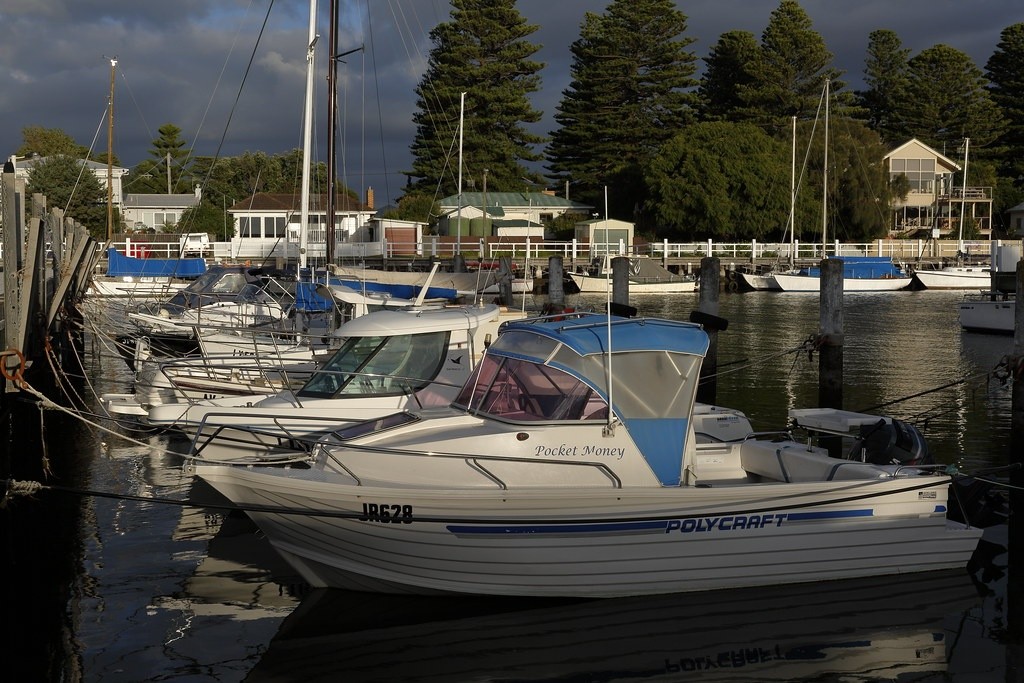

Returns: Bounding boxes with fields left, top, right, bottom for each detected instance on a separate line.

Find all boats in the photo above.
left=567, top=254, right=696, bottom=293
left=958, top=239, right=1021, bottom=335
left=190, top=314, right=984, bottom=601
left=1, top=0, right=759, bottom=455
left=236, top=566, right=983, bottom=683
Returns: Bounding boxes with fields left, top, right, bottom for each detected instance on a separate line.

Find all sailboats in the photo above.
left=773, top=78, right=914, bottom=293
left=912, top=136, right=1011, bottom=290
left=739, top=116, right=854, bottom=292
